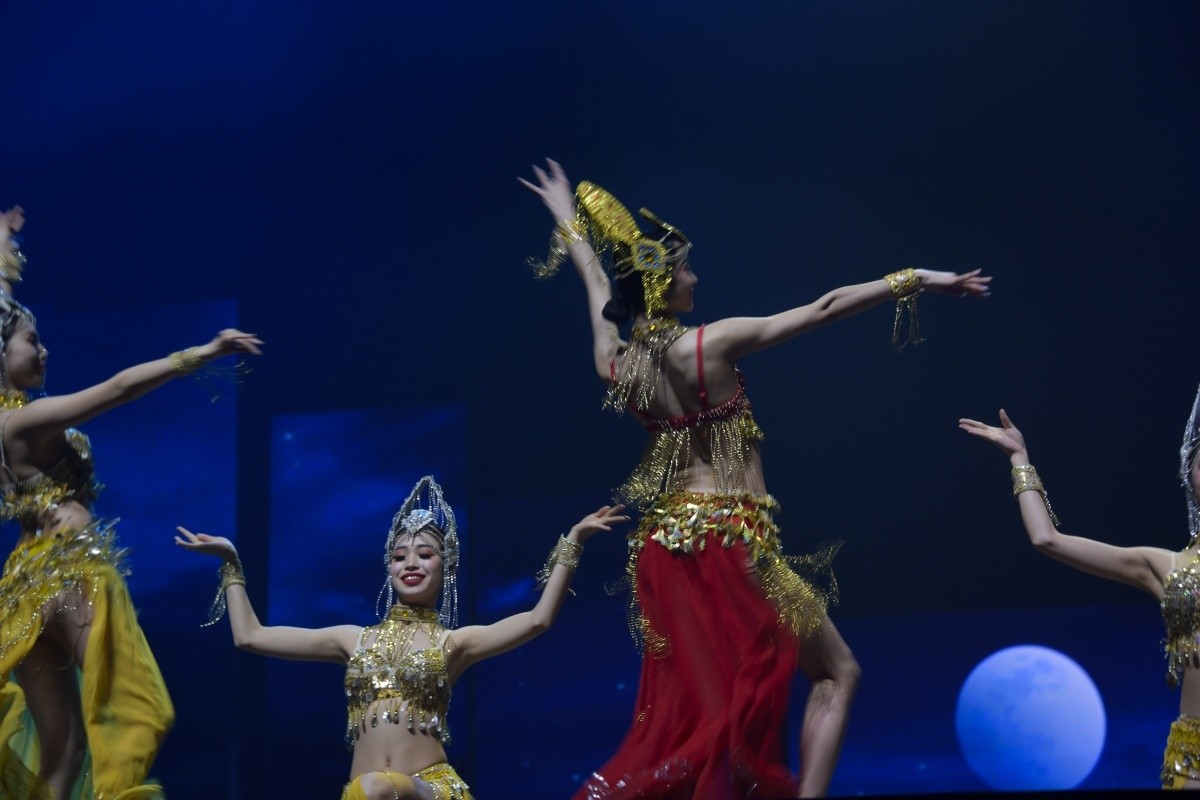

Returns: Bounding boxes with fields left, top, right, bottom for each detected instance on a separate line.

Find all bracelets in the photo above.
left=1011, top=464, right=1045, bottom=501
left=221, top=559, right=246, bottom=588
left=885, top=268, right=920, bottom=300
left=560, top=221, right=587, bottom=246
left=171, top=347, right=203, bottom=376
left=556, top=538, right=584, bottom=571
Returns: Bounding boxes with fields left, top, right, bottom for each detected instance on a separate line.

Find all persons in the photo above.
left=517, top=158, right=992, bottom=800
left=0, top=207, right=261, bottom=800
left=175, top=475, right=631, bottom=800
left=959, top=389, right=1200, bottom=789
